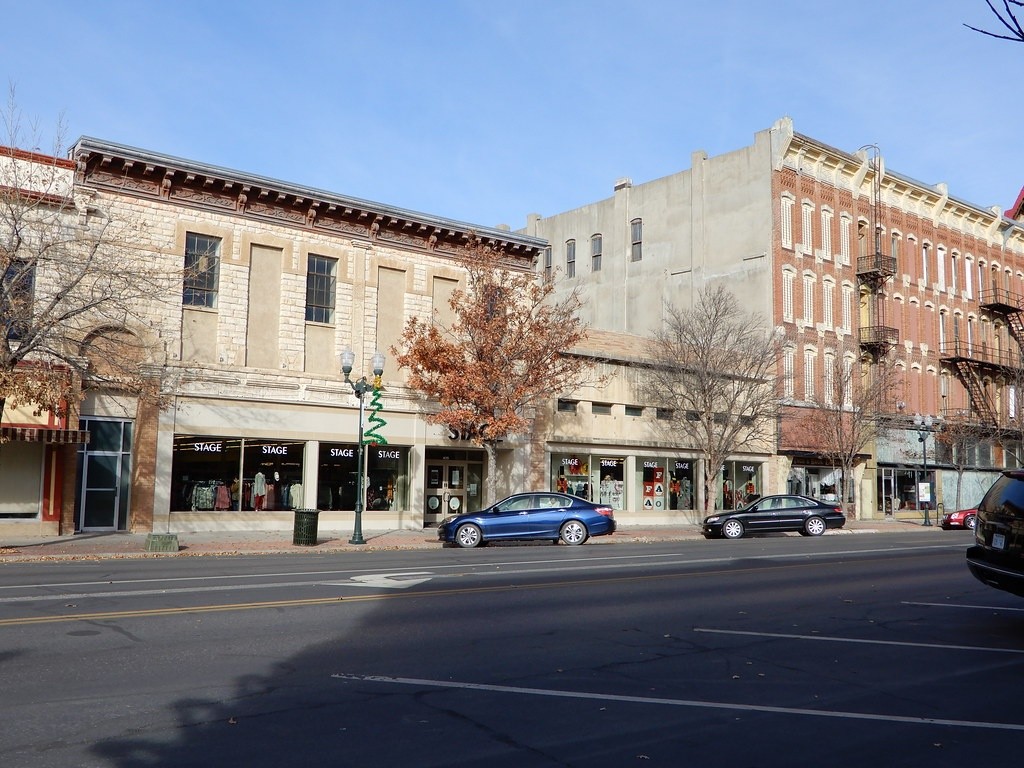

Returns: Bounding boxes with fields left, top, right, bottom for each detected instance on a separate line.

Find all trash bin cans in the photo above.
left=292, top=507, right=322, bottom=547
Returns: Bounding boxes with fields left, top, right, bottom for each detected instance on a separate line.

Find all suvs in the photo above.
left=965, top=470, right=1024, bottom=596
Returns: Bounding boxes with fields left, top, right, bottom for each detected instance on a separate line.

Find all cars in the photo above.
left=700, top=492, right=847, bottom=539
left=943, top=501, right=986, bottom=529
left=437, top=490, right=617, bottom=548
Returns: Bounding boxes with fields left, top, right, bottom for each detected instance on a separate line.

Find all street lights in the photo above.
left=914, top=413, right=937, bottom=527
left=337, top=343, right=387, bottom=544
